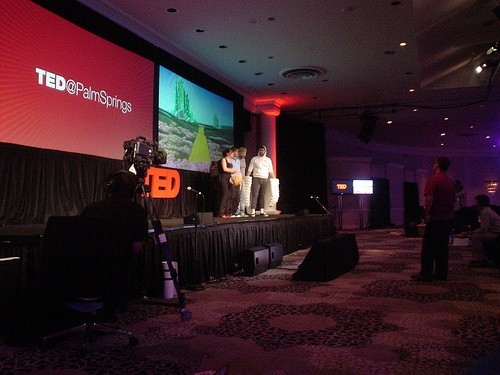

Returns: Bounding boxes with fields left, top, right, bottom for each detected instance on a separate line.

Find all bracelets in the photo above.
left=424, top=212, right=431, bottom=216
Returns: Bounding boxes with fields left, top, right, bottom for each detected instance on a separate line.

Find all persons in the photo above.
left=80, top=170, right=163, bottom=291
left=466, top=194, right=500, bottom=264
left=454, top=180, right=466, bottom=231
left=409, top=155, right=456, bottom=281
left=217, top=145, right=276, bottom=219
left=124, top=136, right=153, bottom=176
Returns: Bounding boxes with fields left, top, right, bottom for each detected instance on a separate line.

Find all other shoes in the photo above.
left=220, top=212, right=268, bottom=218
left=433, top=274, right=447, bottom=281
left=411, top=274, right=432, bottom=281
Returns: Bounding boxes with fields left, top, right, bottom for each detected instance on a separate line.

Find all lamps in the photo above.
left=475, top=61, right=498, bottom=74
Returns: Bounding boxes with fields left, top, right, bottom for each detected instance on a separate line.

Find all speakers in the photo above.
left=262, top=242, right=283, bottom=268
left=292, top=233, right=360, bottom=281
left=243, top=245, right=270, bottom=275
left=45, top=216, right=80, bottom=231
left=183, top=211, right=214, bottom=224
left=295, top=209, right=309, bottom=216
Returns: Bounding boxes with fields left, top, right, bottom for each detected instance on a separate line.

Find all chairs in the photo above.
left=39, top=216, right=149, bottom=358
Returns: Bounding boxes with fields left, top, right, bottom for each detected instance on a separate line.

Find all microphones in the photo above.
left=187, top=186, right=204, bottom=197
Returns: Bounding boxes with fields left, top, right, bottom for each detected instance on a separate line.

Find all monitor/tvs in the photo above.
left=330, top=179, right=353, bottom=195
left=353, top=179, right=374, bottom=195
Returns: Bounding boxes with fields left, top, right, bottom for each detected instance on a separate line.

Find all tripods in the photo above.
left=132, top=185, right=192, bottom=322
